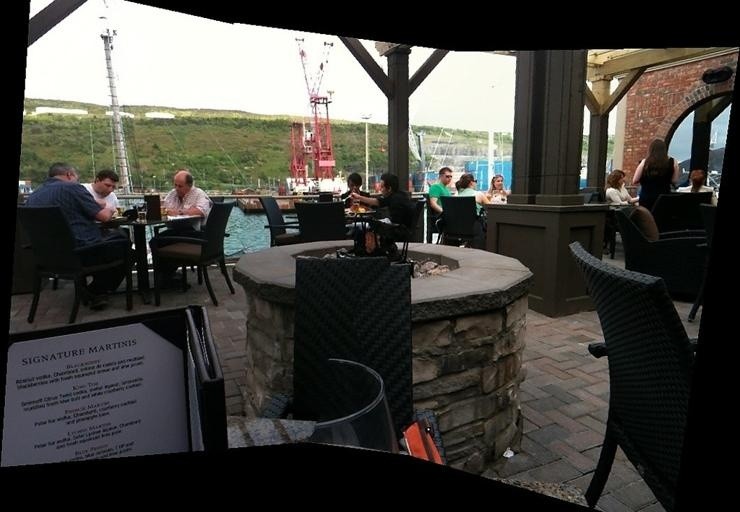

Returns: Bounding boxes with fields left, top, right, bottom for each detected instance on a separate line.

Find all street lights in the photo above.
left=360, top=114, right=373, bottom=194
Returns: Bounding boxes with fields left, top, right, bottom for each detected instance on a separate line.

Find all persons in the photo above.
left=25, top=162, right=137, bottom=311
left=341, top=172, right=370, bottom=208
left=149, top=170, right=217, bottom=293
left=430, top=167, right=510, bottom=249
left=353, top=174, right=415, bottom=242
left=316, top=193, right=354, bottom=210
left=75, top=170, right=132, bottom=247
left=605, top=138, right=718, bottom=211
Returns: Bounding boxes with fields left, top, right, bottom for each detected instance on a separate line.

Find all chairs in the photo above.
left=15, top=197, right=237, bottom=324
left=293, top=255, right=413, bottom=454
left=259, top=194, right=487, bottom=250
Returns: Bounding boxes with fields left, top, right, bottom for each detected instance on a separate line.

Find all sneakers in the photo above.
left=81, top=285, right=112, bottom=311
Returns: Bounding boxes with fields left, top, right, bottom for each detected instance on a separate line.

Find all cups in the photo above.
left=160, top=206, right=167, bottom=221
left=112, top=199, right=146, bottom=223
